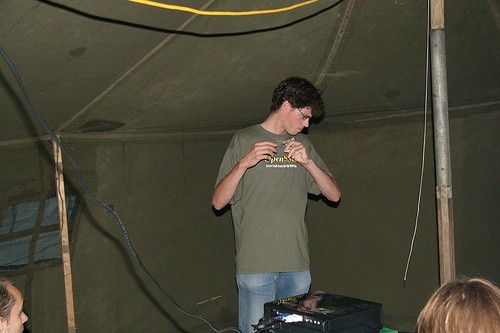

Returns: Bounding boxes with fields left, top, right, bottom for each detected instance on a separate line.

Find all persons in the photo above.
left=416, top=277, right=500, bottom=333
left=0, top=277, right=28, bottom=333
left=212, top=76, right=342, bottom=333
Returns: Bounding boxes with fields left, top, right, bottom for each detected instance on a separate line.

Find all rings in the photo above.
left=291, top=138, right=296, bottom=141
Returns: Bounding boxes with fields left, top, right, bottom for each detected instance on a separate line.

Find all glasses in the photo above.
left=297, top=108, right=313, bottom=120
